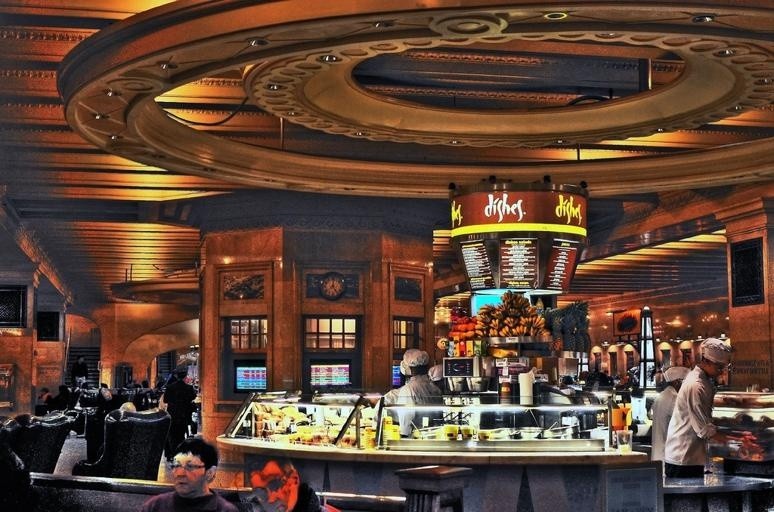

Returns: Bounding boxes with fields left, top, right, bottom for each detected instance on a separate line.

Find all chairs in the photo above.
left=0, top=387, right=172, bottom=481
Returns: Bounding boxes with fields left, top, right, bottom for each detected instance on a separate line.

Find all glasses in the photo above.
left=167, top=463, right=206, bottom=472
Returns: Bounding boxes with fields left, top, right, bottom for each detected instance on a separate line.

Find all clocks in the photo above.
left=317, top=271, right=347, bottom=301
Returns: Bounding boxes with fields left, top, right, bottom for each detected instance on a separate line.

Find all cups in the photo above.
left=519, top=373, right=534, bottom=396
left=476, top=430, right=490, bottom=440
left=444, top=424, right=460, bottom=440
left=500, top=396, right=511, bottom=405
left=520, top=396, right=533, bottom=405
left=500, top=382, right=511, bottom=396
left=460, top=426, right=476, bottom=438
left=617, top=430, right=632, bottom=452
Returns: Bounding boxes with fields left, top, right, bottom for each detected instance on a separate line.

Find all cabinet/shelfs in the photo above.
left=222, top=390, right=662, bottom=452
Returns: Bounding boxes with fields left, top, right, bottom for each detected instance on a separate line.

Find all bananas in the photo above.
left=478, top=289, right=550, bottom=337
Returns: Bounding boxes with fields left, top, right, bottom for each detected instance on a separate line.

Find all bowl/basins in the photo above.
left=446, top=377, right=466, bottom=392
left=487, top=425, right=579, bottom=439
left=466, top=376, right=490, bottom=391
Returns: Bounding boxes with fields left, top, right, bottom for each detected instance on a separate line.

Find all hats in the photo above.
left=664, top=366, right=692, bottom=382
left=701, top=338, right=730, bottom=365
left=400, top=349, right=443, bottom=381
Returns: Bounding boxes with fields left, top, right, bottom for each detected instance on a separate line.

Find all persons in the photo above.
left=373, top=348, right=443, bottom=428
left=663, top=337, right=734, bottom=478
left=241, top=452, right=327, bottom=512
left=163, top=367, right=196, bottom=461
left=36, top=387, right=54, bottom=404
left=651, top=366, right=692, bottom=461
left=71, top=356, right=88, bottom=388
left=137, top=438, right=239, bottom=512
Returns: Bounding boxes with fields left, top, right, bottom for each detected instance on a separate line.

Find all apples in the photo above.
left=445, top=315, right=475, bottom=341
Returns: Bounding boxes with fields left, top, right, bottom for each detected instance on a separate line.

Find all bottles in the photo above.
left=535, top=297, right=544, bottom=317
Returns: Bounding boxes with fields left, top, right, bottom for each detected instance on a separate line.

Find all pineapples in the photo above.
left=539, top=299, right=593, bottom=352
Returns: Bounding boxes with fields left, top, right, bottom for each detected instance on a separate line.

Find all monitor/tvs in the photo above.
left=442, top=357, right=475, bottom=378
left=308, top=359, right=352, bottom=388
left=233, top=358, right=267, bottom=394
left=391, top=364, right=402, bottom=389
